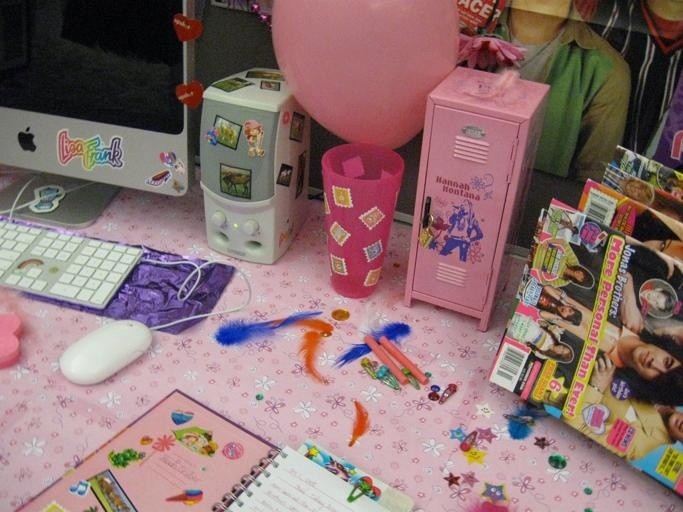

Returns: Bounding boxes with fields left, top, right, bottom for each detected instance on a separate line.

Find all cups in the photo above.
left=321, top=145, right=405, bottom=300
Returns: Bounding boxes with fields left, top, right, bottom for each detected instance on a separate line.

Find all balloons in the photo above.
left=273, top=0, right=462, bottom=148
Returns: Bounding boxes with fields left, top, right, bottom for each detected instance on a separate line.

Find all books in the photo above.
left=489, top=145, right=683, bottom=497
left=13, top=388, right=415, bottom=512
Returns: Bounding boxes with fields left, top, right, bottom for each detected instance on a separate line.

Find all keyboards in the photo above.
left=0, top=220, right=142, bottom=311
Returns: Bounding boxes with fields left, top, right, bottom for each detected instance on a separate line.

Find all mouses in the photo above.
left=59, top=318, right=154, bottom=387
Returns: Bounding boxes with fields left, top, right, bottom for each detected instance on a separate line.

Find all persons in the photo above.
left=243, top=120, right=265, bottom=159
left=483, top=0, right=632, bottom=184
left=439, top=200, right=483, bottom=261
left=578, top=0, right=683, bottom=171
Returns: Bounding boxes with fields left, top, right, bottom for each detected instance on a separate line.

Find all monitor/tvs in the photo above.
left=1, top=0, right=197, bottom=230
